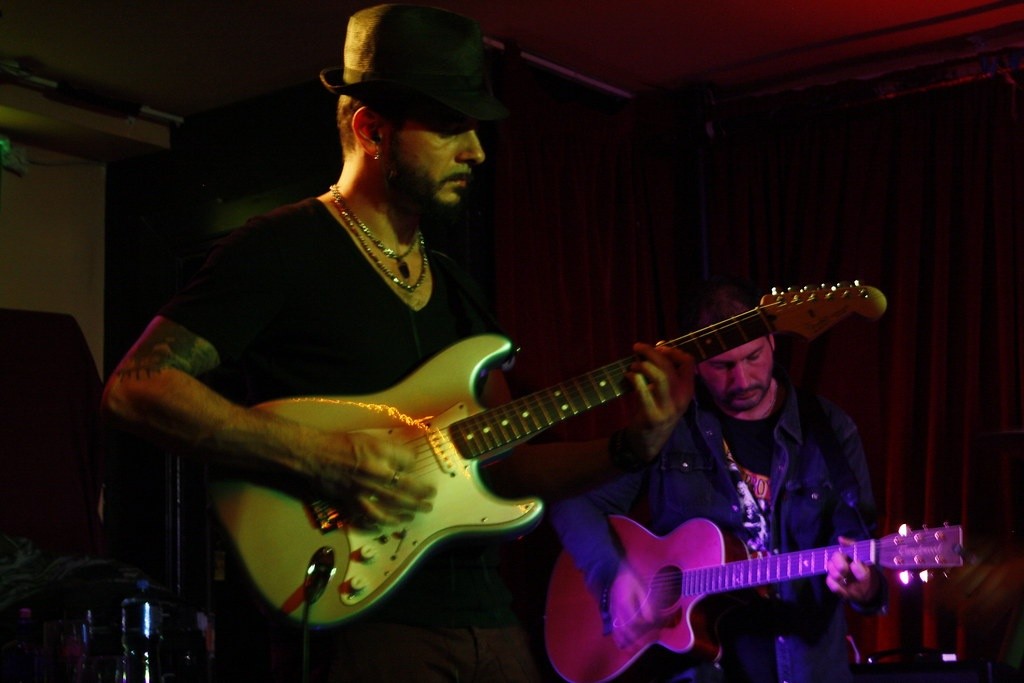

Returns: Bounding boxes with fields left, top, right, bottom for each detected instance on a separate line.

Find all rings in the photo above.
left=391, top=471, right=400, bottom=485
left=843, top=577, right=848, bottom=585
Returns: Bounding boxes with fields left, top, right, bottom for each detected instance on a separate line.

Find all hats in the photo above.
left=319, top=3, right=511, bottom=121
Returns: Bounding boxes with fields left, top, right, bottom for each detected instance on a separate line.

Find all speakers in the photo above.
left=846, top=660, right=1016, bottom=683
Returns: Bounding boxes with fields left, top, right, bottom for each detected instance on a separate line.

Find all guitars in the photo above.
left=205, top=280, right=891, bottom=628
left=542, top=506, right=966, bottom=683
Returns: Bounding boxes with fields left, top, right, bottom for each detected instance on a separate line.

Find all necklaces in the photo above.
left=330, top=184, right=428, bottom=292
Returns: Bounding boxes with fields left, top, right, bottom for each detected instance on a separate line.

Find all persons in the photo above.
left=549, top=272, right=890, bottom=683
left=101, top=0, right=693, bottom=683
